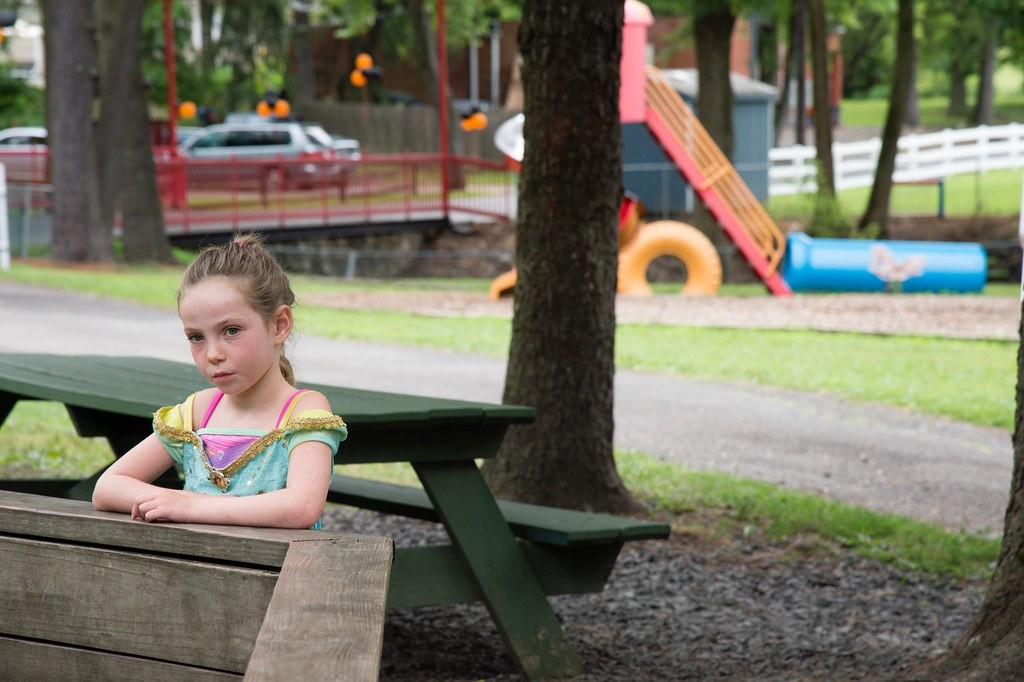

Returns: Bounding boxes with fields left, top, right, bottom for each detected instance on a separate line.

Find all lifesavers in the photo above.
left=616, top=220, right=723, bottom=300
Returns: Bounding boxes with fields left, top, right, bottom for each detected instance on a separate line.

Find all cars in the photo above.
left=146, top=122, right=205, bottom=164
left=295, top=124, right=363, bottom=197
left=151, top=121, right=339, bottom=194
left=0, top=124, right=56, bottom=184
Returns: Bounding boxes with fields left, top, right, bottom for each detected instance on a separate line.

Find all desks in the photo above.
left=0, top=354, right=673, bottom=682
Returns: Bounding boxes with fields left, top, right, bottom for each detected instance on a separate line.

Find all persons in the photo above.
left=85, top=237, right=348, bottom=524
